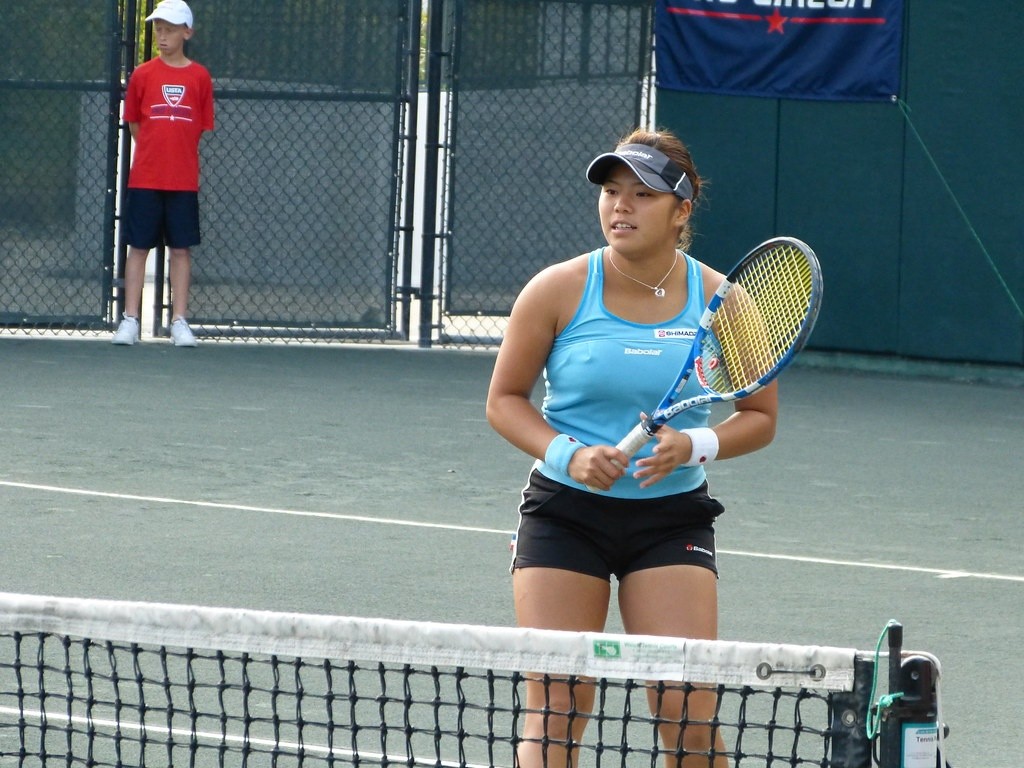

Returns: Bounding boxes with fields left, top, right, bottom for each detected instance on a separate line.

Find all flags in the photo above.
left=653, top=1, right=903, bottom=104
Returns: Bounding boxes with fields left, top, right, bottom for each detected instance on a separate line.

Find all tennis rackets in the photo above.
left=581, top=235, right=825, bottom=494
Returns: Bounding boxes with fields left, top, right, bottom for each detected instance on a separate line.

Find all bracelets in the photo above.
left=545, top=433, right=587, bottom=477
left=679, top=427, right=719, bottom=467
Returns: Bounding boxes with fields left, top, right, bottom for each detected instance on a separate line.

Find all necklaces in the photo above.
left=609, top=251, right=677, bottom=297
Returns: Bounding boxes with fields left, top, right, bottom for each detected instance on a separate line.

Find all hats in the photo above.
left=586, top=144, right=695, bottom=201
left=145, top=0, right=193, bottom=29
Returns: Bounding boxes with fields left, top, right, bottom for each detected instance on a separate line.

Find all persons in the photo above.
left=111, top=0, right=217, bottom=348
left=485, top=130, right=778, bottom=768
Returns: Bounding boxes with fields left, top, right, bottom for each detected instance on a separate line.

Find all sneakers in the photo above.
left=169, top=315, right=197, bottom=348
left=111, top=312, right=139, bottom=345
